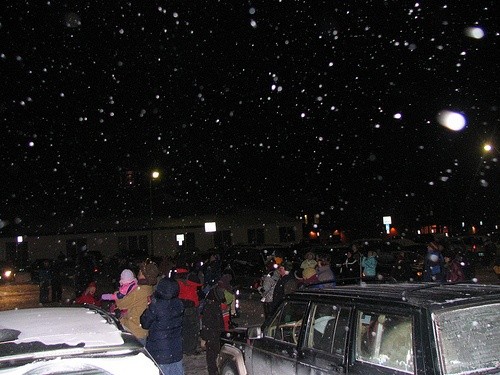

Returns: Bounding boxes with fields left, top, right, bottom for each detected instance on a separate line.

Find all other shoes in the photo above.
left=183, top=350, right=200, bottom=356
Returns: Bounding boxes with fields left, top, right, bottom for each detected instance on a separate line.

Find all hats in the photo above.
left=176, top=268, right=188, bottom=273
left=428, top=241, right=439, bottom=251
left=305, top=252, right=315, bottom=259
left=280, top=260, right=293, bottom=271
left=209, top=288, right=226, bottom=303
left=274, top=257, right=283, bottom=264
left=141, top=263, right=159, bottom=280
left=119, top=269, right=136, bottom=284
left=155, top=278, right=179, bottom=298
left=266, top=262, right=275, bottom=269
left=89, top=282, right=96, bottom=287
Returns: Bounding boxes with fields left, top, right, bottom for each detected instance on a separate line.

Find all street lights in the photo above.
left=149, top=171, right=159, bottom=256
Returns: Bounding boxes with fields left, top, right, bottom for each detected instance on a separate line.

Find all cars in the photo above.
left=221, top=234, right=444, bottom=292
left=217, top=280, right=500, bottom=375
left=0, top=303, right=163, bottom=375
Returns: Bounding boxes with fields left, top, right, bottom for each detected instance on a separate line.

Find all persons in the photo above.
left=140, top=278, right=184, bottom=375
left=39, top=233, right=500, bottom=375
